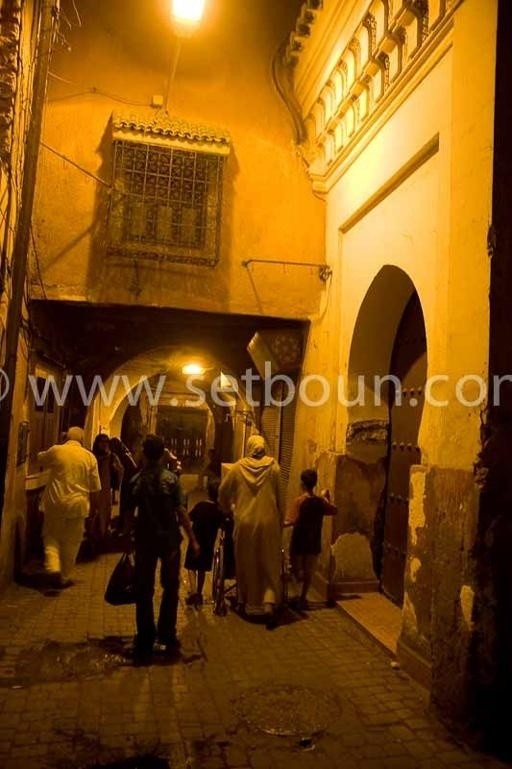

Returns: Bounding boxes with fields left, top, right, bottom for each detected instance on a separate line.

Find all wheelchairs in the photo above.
left=212, top=528, right=288, bottom=614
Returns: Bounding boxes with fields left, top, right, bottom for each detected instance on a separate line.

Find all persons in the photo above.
left=283, top=470, right=339, bottom=608
left=36, top=426, right=224, bottom=647
left=219, top=434, right=291, bottom=621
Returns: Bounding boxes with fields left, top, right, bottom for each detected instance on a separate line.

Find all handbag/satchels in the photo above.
left=104, top=552, right=135, bottom=605
